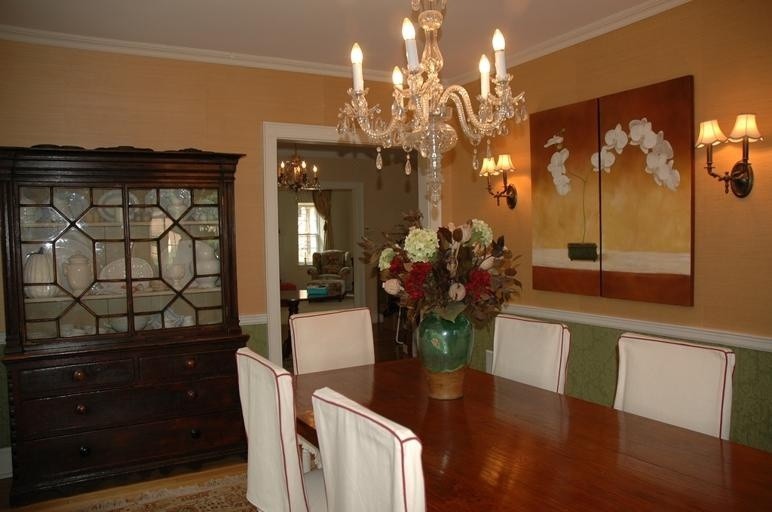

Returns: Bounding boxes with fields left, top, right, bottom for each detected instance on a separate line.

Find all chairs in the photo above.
left=235, top=347, right=329, bottom=512
left=489, top=314, right=572, bottom=397
left=289, top=307, right=381, bottom=472
left=613, top=331, right=738, bottom=441
left=311, top=383, right=428, bottom=512
left=307, top=249, right=353, bottom=300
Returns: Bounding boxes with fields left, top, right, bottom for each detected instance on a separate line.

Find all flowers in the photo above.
left=354, top=205, right=522, bottom=331
left=542, top=113, right=682, bottom=243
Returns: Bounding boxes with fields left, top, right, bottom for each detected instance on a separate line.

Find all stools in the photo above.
left=307, top=280, right=347, bottom=304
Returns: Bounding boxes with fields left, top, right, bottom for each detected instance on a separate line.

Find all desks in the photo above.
left=281, top=286, right=346, bottom=359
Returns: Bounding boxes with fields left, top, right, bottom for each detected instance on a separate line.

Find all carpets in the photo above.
left=68, top=470, right=258, bottom=512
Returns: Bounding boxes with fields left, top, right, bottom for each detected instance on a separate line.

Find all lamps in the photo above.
left=335, top=2, right=530, bottom=180
left=696, top=114, right=765, bottom=198
left=477, top=154, right=522, bottom=210
left=278, top=144, right=322, bottom=198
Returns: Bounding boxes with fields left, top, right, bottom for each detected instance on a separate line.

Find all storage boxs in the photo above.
left=309, top=288, right=329, bottom=297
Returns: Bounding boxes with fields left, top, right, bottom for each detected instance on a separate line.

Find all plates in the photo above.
left=99, top=258, right=153, bottom=295
left=159, top=239, right=219, bottom=289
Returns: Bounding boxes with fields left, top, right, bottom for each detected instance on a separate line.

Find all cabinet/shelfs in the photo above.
left=2, top=142, right=250, bottom=503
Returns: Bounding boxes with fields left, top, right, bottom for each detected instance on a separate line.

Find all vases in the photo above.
left=23, top=251, right=63, bottom=299
left=565, top=240, right=601, bottom=262
left=417, top=316, right=477, bottom=401
